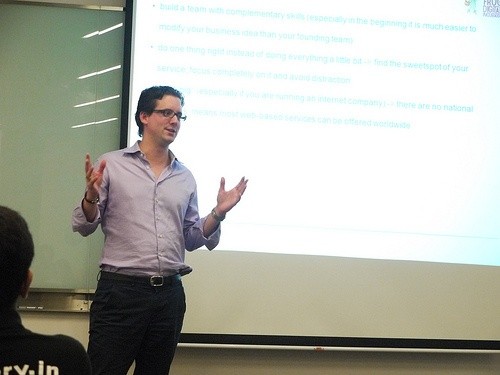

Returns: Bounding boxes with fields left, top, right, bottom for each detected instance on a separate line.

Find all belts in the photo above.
left=100, top=272, right=181, bottom=287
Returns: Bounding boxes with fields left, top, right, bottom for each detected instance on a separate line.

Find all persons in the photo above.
left=0, top=206, right=91, bottom=375
left=71, top=87, right=248, bottom=375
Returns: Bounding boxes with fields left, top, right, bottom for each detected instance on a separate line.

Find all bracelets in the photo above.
left=84, top=191, right=99, bottom=204
left=211, top=208, right=225, bottom=221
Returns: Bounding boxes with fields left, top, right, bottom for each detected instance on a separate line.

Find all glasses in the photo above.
left=153, top=109, right=182, bottom=120
